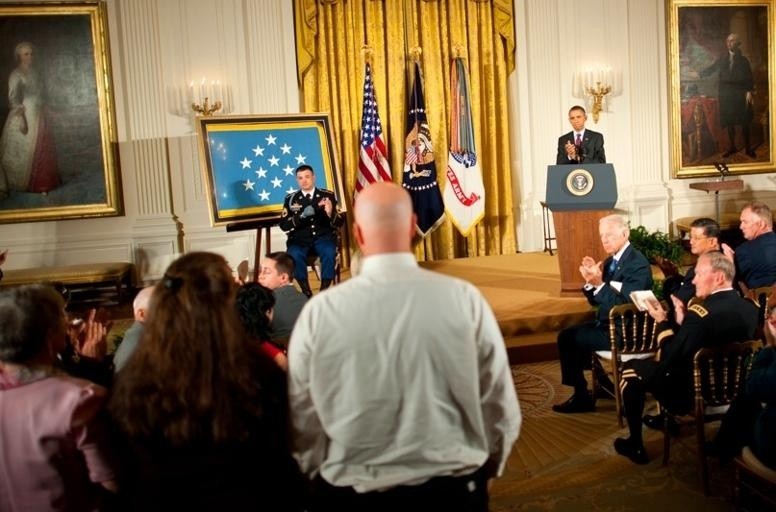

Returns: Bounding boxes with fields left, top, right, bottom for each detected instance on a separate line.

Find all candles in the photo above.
left=581, top=66, right=617, bottom=94
left=190, top=79, right=223, bottom=108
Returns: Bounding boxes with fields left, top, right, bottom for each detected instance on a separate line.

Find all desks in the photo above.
left=680, top=93, right=721, bottom=167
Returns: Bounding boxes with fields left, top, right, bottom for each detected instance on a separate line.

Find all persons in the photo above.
left=280, top=165, right=341, bottom=298
left=101, top=252, right=320, bottom=508
left=34, top=251, right=310, bottom=389
left=553, top=213, right=654, bottom=414
left=690, top=33, right=762, bottom=160
left=288, top=183, right=524, bottom=509
left=0, top=286, right=120, bottom=508
left=2, top=43, right=59, bottom=193
left=613, top=202, right=775, bottom=466
left=0, top=248, right=10, bottom=276
left=712, top=284, right=775, bottom=508
left=555, top=106, right=606, bottom=165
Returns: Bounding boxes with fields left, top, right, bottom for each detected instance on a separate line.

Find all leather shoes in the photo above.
left=614, top=438, right=650, bottom=464
left=643, top=414, right=664, bottom=431
left=552, top=394, right=596, bottom=412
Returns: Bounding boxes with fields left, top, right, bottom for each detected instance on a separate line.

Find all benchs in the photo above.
left=674, top=212, right=776, bottom=240
left=0, top=261, right=132, bottom=308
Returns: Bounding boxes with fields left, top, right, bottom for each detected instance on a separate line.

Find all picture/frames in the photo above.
left=664, top=2, right=776, bottom=180
left=194, top=112, right=350, bottom=228
left=0, top=1, right=126, bottom=225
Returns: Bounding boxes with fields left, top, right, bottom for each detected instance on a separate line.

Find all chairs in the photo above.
left=591, top=297, right=671, bottom=429
left=658, top=337, right=766, bottom=498
left=745, top=284, right=776, bottom=343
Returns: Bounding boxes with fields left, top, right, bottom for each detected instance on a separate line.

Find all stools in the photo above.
left=539, top=199, right=557, bottom=254
left=733, top=446, right=776, bottom=512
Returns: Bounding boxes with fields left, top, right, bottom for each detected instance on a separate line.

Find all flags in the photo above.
left=440, top=59, right=486, bottom=237
left=404, top=61, right=448, bottom=237
left=353, top=57, right=395, bottom=189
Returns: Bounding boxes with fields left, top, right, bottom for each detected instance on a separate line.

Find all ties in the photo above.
left=608, top=259, right=616, bottom=273
left=576, top=134, right=582, bottom=147
left=306, top=194, right=310, bottom=200
